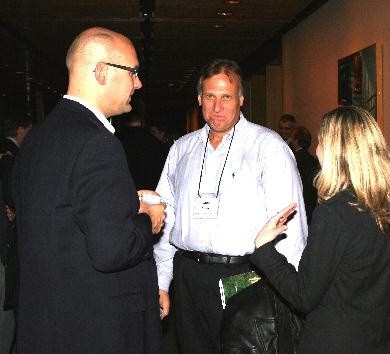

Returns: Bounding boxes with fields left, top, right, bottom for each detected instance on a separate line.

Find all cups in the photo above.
left=141, top=193, right=162, bottom=205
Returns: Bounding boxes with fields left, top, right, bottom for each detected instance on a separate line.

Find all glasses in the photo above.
left=93, top=63, right=141, bottom=79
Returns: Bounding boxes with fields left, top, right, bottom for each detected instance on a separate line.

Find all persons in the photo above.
left=11, top=26, right=167, bottom=354
left=250, top=107, right=390, bottom=354
left=0, top=98, right=320, bottom=312
left=150, top=60, right=308, bottom=354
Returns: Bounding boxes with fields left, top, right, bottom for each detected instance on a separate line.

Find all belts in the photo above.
left=180, top=250, right=249, bottom=263
